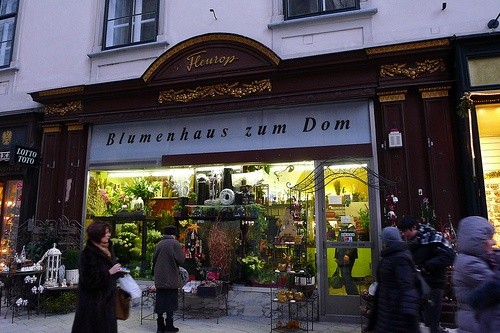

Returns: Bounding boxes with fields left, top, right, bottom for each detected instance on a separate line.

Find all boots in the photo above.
left=164, top=318, right=179, bottom=333
left=157, top=317, right=166, bottom=333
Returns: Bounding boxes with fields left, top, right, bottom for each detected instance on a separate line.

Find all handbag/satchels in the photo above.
left=330, top=267, right=340, bottom=289
left=119, top=274, right=142, bottom=299
left=416, top=275, right=431, bottom=300
left=116, top=286, right=132, bottom=320
left=179, top=266, right=189, bottom=286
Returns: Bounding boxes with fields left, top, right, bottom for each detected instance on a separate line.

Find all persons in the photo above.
left=364, top=226, right=424, bottom=333
left=71, top=220, right=131, bottom=333
left=399, top=220, right=497, bottom=333
left=334, top=215, right=359, bottom=295
left=452, top=216, right=500, bottom=333
left=154, top=226, right=186, bottom=333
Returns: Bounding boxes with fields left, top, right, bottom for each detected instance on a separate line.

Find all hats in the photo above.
left=164, top=226, right=179, bottom=235
left=380, top=227, right=404, bottom=242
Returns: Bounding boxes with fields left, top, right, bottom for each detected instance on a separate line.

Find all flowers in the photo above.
left=88, top=168, right=164, bottom=278
left=241, top=256, right=267, bottom=274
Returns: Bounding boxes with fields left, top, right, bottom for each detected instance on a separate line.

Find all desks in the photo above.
left=39, top=282, right=79, bottom=319
left=90, top=214, right=162, bottom=276
left=0, top=265, right=47, bottom=324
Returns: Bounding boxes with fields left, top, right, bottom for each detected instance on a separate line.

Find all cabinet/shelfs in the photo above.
left=268, top=241, right=320, bottom=333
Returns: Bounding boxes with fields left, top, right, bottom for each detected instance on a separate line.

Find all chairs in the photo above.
left=3, top=262, right=47, bottom=319
